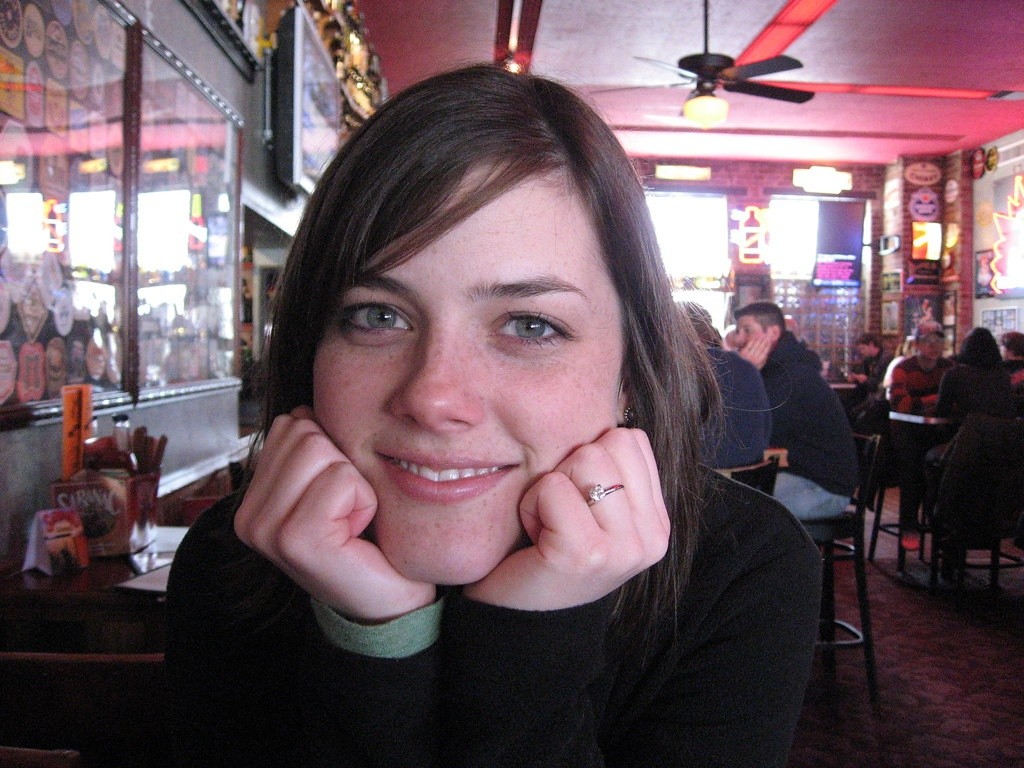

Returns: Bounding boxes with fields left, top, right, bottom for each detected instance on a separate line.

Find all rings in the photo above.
left=587, top=484, right=623, bottom=506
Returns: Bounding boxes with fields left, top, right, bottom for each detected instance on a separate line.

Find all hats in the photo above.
left=916, top=322, right=946, bottom=339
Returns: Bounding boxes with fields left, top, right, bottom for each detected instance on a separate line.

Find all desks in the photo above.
left=0, top=526, right=190, bottom=617
left=828, top=383, right=858, bottom=392
left=887, top=407, right=955, bottom=573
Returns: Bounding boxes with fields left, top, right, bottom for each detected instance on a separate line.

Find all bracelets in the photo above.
left=315, top=597, right=446, bottom=659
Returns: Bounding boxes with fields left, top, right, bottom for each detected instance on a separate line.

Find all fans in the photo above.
left=683, top=0, right=728, bottom=131
left=589, top=0, right=816, bottom=105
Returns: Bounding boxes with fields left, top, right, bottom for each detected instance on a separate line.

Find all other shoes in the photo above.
left=899, top=530, right=921, bottom=551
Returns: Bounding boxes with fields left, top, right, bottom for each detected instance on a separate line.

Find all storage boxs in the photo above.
left=48, top=471, right=160, bottom=553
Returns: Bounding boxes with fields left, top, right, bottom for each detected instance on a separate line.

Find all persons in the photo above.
left=846, top=332, right=894, bottom=416
left=881, top=321, right=1024, bottom=552
left=163, top=61, right=822, bottom=767
left=680, top=301, right=771, bottom=470
left=723, top=301, right=858, bottom=520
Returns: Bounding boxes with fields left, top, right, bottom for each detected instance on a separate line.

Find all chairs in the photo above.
left=0, top=649, right=165, bottom=768
left=725, top=387, right=1024, bottom=710
